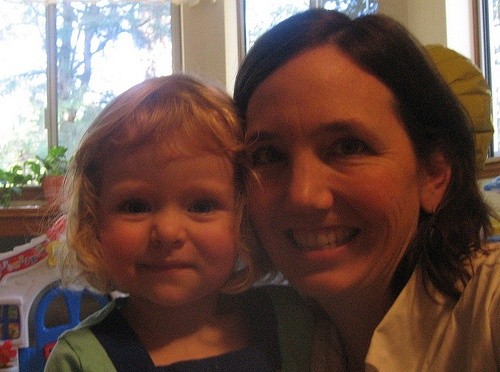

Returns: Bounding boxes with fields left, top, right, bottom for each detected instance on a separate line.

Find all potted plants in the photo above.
left=0, top=144, right=69, bottom=208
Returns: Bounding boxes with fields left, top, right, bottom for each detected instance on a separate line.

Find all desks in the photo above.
left=0, top=200, right=56, bottom=239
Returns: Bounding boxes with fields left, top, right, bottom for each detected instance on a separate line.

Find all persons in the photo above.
left=233, top=5, right=500, bottom=372
left=41, top=74, right=349, bottom=372
